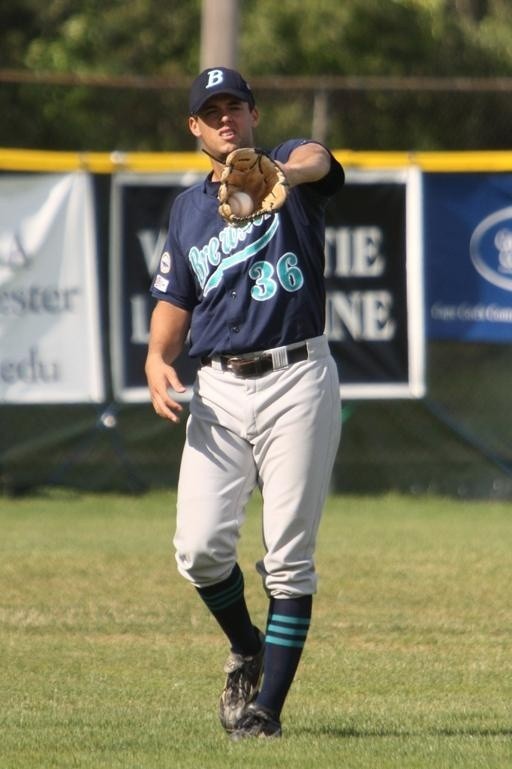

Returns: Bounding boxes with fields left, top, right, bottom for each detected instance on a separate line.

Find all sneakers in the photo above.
left=216, top=623, right=266, bottom=733
left=226, top=704, right=282, bottom=740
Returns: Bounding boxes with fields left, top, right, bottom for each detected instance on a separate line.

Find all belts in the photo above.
left=200, top=343, right=308, bottom=379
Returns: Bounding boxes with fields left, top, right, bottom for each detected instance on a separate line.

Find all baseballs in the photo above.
left=228, top=193, right=255, bottom=217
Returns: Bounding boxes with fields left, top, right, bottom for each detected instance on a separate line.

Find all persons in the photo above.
left=145, top=65, right=346, bottom=744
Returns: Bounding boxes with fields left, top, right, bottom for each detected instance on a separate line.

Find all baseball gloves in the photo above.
left=216, top=145, right=290, bottom=227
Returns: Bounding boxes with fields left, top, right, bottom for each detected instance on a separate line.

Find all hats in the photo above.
left=187, top=66, right=255, bottom=114
